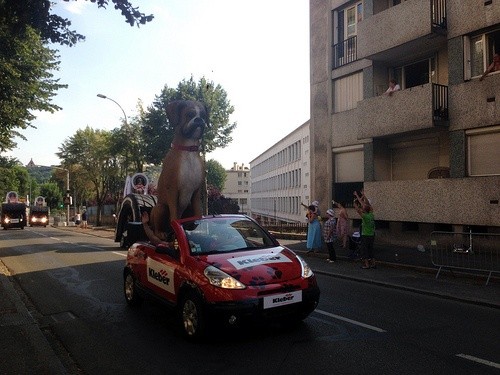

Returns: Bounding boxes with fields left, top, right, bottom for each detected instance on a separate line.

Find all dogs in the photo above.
left=149, top=99, right=208, bottom=241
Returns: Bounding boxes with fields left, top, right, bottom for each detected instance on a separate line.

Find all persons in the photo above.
left=384, top=80, right=401, bottom=96
left=353, top=191, right=377, bottom=269
left=74, top=212, right=81, bottom=231
left=323, top=209, right=337, bottom=263
left=301, top=200, right=323, bottom=256
left=80, top=211, right=89, bottom=231
left=332, top=200, right=348, bottom=249
left=142, top=211, right=195, bottom=250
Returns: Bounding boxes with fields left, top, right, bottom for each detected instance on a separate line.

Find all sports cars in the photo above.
left=122, top=213, right=321, bottom=339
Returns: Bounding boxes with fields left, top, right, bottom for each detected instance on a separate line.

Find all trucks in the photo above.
left=1, top=201, right=27, bottom=230
left=30, top=205, right=52, bottom=225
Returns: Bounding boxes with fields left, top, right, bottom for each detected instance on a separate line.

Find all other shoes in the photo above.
left=329, top=260, right=335, bottom=263
left=314, top=250, right=320, bottom=253
left=360, top=266, right=369, bottom=269
left=369, top=266, right=376, bottom=269
left=338, top=244, right=346, bottom=248
left=326, top=259, right=331, bottom=261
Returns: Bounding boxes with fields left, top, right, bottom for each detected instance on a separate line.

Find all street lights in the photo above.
left=97, top=93, right=129, bottom=178
left=52, top=165, right=73, bottom=226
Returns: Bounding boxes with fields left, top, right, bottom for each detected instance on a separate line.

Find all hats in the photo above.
left=312, top=201, right=319, bottom=206
left=327, top=209, right=335, bottom=217
left=352, top=232, right=361, bottom=238
